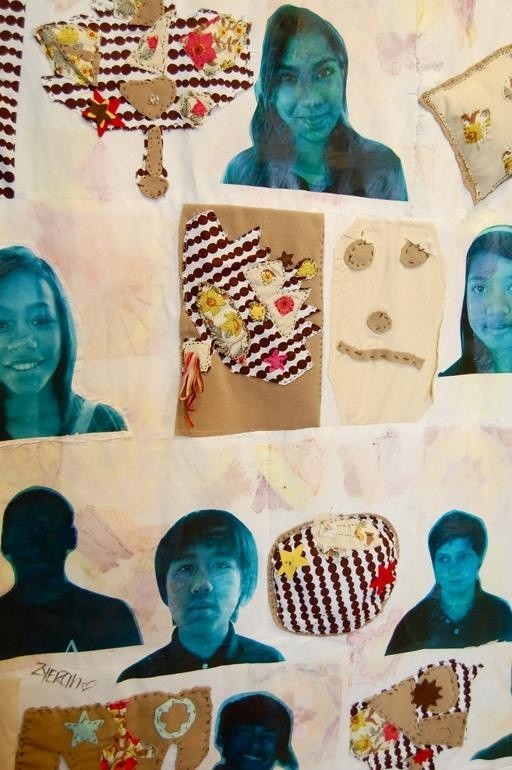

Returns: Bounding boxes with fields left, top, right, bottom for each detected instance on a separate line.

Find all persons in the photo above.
left=116, top=509, right=286, bottom=682
left=385, top=508, right=511, bottom=656
left=209, top=691, right=299, bottom=770
left=0, top=486, right=144, bottom=659
left=438, top=225, right=512, bottom=374
left=222, top=3, right=409, bottom=202
left=0, top=244, right=127, bottom=442
